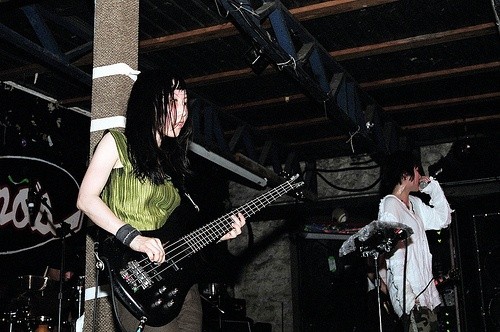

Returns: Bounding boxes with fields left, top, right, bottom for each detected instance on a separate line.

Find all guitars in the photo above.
left=97, top=173, right=304, bottom=327
left=434, top=267, right=462, bottom=286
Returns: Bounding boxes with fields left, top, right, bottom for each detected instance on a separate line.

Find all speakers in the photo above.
left=470, top=212, right=500, bottom=332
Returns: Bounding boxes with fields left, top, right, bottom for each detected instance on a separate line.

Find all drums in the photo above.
left=26, top=316, right=54, bottom=332
left=57, top=320, right=75, bottom=332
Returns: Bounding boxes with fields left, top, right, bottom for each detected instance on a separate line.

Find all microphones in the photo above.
left=31, top=188, right=47, bottom=201
left=420, top=168, right=443, bottom=189
